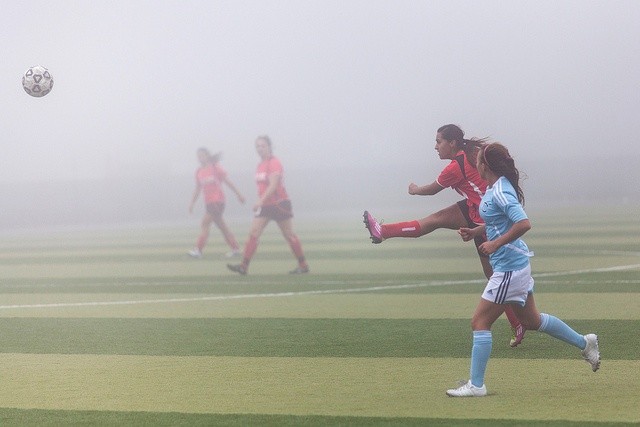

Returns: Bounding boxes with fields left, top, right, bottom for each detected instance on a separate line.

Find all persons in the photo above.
left=445, top=145, right=600, bottom=397
left=363, top=125, right=525, bottom=347
left=226, top=135, right=310, bottom=277
left=187, top=147, right=246, bottom=257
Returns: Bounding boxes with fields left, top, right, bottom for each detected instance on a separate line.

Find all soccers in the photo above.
left=23, top=66, right=53, bottom=97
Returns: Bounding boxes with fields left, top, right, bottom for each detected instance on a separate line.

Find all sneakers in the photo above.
left=226, top=262, right=247, bottom=275
left=363, top=211, right=385, bottom=244
left=289, top=264, right=309, bottom=274
left=580, top=334, right=601, bottom=373
left=224, top=248, right=242, bottom=258
left=509, top=323, right=526, bottom=347
left=187, top=247, right=203, bottom=258
left=445, top=381, right=487, bottom=398
